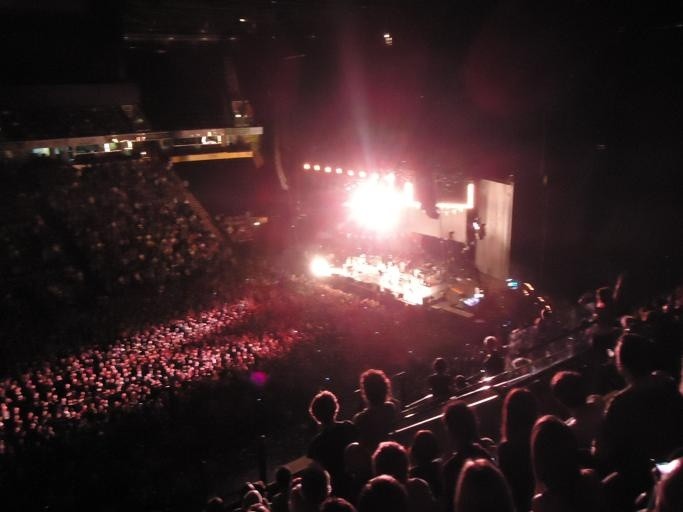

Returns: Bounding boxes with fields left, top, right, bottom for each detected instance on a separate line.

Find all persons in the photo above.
left=0, top=159, right=683, bottom=512
